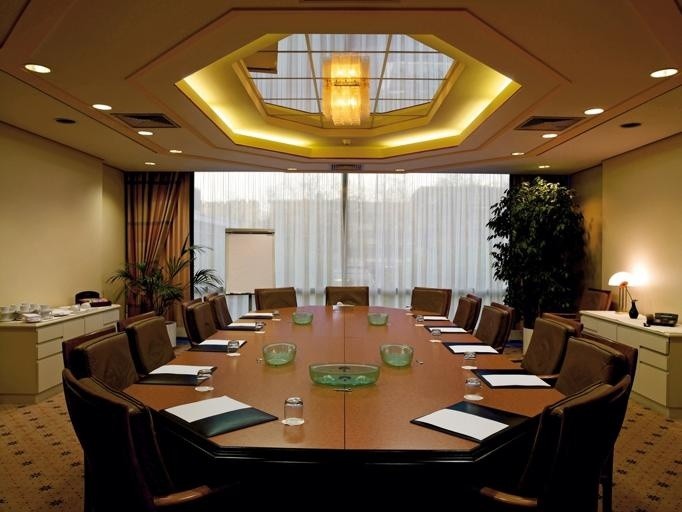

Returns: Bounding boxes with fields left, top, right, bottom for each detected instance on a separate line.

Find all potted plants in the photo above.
left=483, top=172, right=596, bottom=358
left=102, top=230, right=227, bottom=350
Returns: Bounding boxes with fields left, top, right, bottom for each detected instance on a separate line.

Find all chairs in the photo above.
left=181, top=298, right=219, bottom=346
left=116, top=309, right=178, bottom=376
left=203, top=292, right=232, bottom=328
left=255, top=286, right=297, bottom=310
left=410, top=286, right=453, bottom=320
left=478, top=330, right=639, bottom=512
left=510, top=310, right=584, bottom=389
left=325, top=285, right=370, bottom=307
left=59, top=366, right=224, bottom=512
left=452, top=292, right=482, bottom=335
left=60, top=325, right=139, bottom=393
left=474, top=301, right=516, bottom=353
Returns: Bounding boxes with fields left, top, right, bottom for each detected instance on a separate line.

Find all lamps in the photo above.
left=319, top=51, right=373, bottom=130
left=608, top=268, right=643, bottom=316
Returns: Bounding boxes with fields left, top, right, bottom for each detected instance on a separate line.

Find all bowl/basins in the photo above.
left=367, top=312, right=387, bottom=327
left=377, top=343, right=415, bottom=367
left=262, top=342, right=296, bottom=366
left=293, top=310, right=315, bottom=325
left=307, top=361, right=380, bottom=385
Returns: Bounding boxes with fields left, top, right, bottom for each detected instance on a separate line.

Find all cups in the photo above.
left=462, top=378, right=483, bottom=400
left=271, top=309, right=280, bottom=321
left=430, top=329, right=442, bottom=343
left=404, top=305, right=414, bottom=315
left=284, top=396, right=304, bottom=426
left=81, top=302, right=92, bottom=309
left=255, top=320, right=264, bottom=332
left=0, top=303, right=51, bottom=322
left=415, top=315, right=424, bottom=327
left=196, top=367, right=214, bottom=390
left=462, top=352, right=476, bottom=369
left=645, top=313, right=653, bottom=325
left=71, top=303, right=80, bottom=311
left=227, top=340, right=240, bottom=354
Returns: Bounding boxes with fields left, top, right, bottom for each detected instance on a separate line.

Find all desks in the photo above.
left=122, top=305, right=570, bottom=512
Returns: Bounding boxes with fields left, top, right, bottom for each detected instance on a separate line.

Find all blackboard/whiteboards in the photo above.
left=225, top=227, right=276, bottom=295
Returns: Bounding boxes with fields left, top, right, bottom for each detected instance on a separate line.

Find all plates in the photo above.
left=50, top=308, right=69, bottom=317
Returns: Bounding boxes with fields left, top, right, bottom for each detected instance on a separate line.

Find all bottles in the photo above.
left=667, top=318, right=674, bottom=324
left=655, top=317, right=661, bottom=322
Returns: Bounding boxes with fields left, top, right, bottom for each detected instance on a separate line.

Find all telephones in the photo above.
left=647, top=313, right=678, bottom=327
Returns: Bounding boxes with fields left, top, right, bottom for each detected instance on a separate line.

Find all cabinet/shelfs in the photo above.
left=578, top=309, right=682, bottom=422
left=1, top=303, right=122, bottom=408
